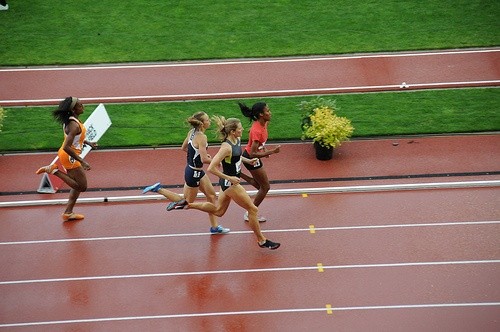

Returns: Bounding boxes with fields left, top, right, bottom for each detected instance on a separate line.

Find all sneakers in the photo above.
left=167, top=199, right=188, bottom=212
left=256, top=238, right=281, bottom=250
left=62, top=212, right=85, bottom=221
left=143, top=181, right=161, bottom=194
left=210, top=225, right=230, bottom=235
left=244, top=211, right=267, bottom=222
left=36, top=164, right=59, bottom=174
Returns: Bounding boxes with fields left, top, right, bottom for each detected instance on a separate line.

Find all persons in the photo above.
left=143, top=112, right=230, bottom=234
left=166, top=115, right=281, bottom=250
left=238, top=101, right=280, bottom=222
left=36, top=96, right=98, bottom=221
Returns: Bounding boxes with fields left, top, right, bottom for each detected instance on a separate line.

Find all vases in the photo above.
left=314, top=140, right=334, bottom=161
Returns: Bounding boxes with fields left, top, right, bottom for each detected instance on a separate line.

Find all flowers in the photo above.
left=301, top=107, right=354, bottom=149
